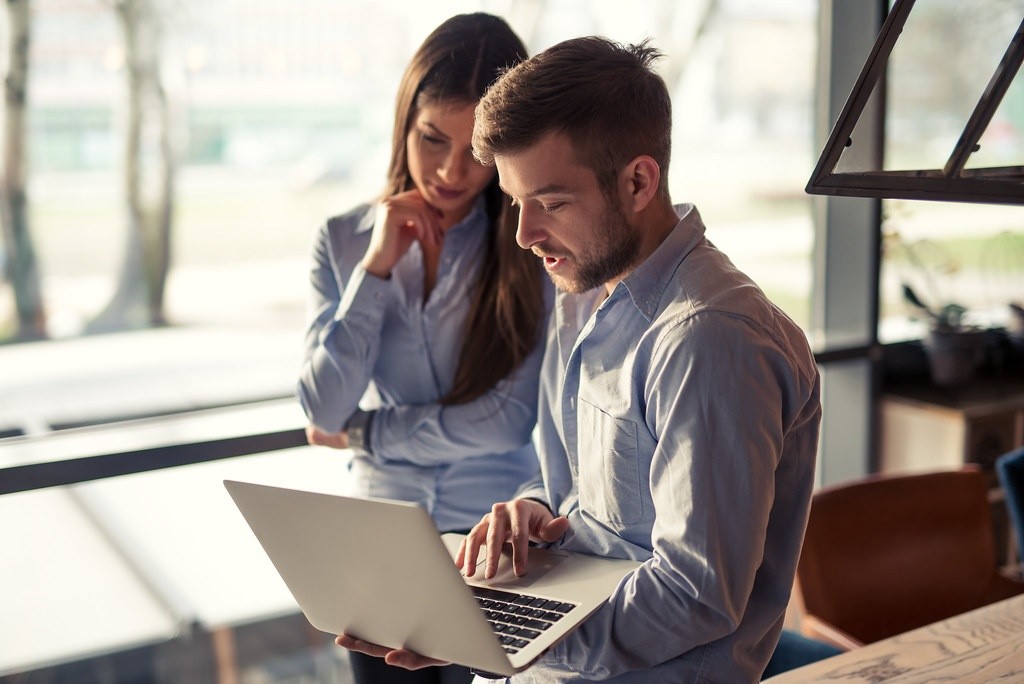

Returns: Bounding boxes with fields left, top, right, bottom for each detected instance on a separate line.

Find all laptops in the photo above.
left=223, top=480, right=645, bottom=677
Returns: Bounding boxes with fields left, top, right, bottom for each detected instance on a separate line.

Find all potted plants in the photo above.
left=904, top=281, right=980, bottom=389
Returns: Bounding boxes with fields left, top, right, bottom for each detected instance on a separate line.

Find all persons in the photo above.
left=335, top=36, right=821, bottom=684
left=292, top=11, right=558, bottom=684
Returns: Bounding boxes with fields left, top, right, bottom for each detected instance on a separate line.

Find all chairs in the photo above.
left=760, top=448, right=1024, bottom=680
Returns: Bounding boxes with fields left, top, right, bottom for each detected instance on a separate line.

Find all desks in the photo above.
left=879, top=393, right=1024, bottom=570
left=759, top=593, right=1024, bottom=684
